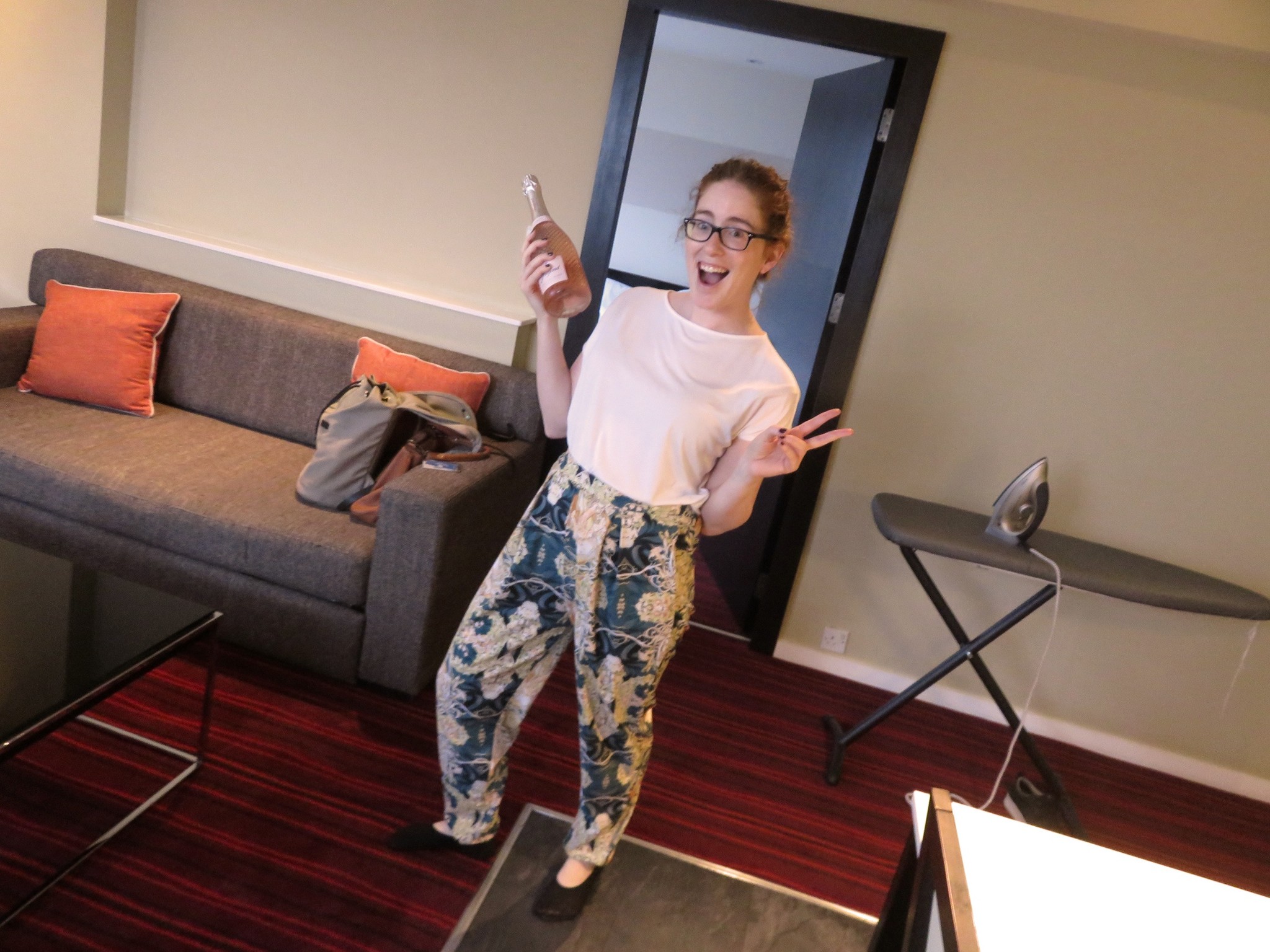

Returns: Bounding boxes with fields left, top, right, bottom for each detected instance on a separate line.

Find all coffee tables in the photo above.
left=0, top=540, right=224, bottom=930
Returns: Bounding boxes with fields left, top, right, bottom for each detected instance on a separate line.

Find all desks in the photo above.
left=868, top=786, right=1270, bottom=952
left=820, top=487, right=1270, bottom=836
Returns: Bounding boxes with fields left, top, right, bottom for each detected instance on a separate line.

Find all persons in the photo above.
left=388, top=160, right=853, bottom=920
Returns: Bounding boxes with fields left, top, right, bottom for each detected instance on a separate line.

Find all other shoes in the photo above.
left=389, top=821, right=503, bottom=860
left=536, top=856, right=603, bottom=919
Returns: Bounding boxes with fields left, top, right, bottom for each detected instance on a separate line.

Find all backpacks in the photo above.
left=297, top=372, right=484, bottom=511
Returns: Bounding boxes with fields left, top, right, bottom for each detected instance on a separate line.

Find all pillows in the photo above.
left=18, top=278, right=181, bottom=419
left=341, top=337, right=489, bottom=417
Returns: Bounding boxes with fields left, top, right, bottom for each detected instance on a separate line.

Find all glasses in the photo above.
left=684, top=217, right=779, bottom=252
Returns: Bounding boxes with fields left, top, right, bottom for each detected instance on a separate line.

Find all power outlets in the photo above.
left=821, top=626, right=850, bottom=654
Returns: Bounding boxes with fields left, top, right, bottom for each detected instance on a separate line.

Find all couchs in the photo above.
left=0, top=249, right=548, bottom=698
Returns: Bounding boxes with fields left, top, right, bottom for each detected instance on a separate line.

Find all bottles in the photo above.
left=519, top=173, right=592, bottom=316
